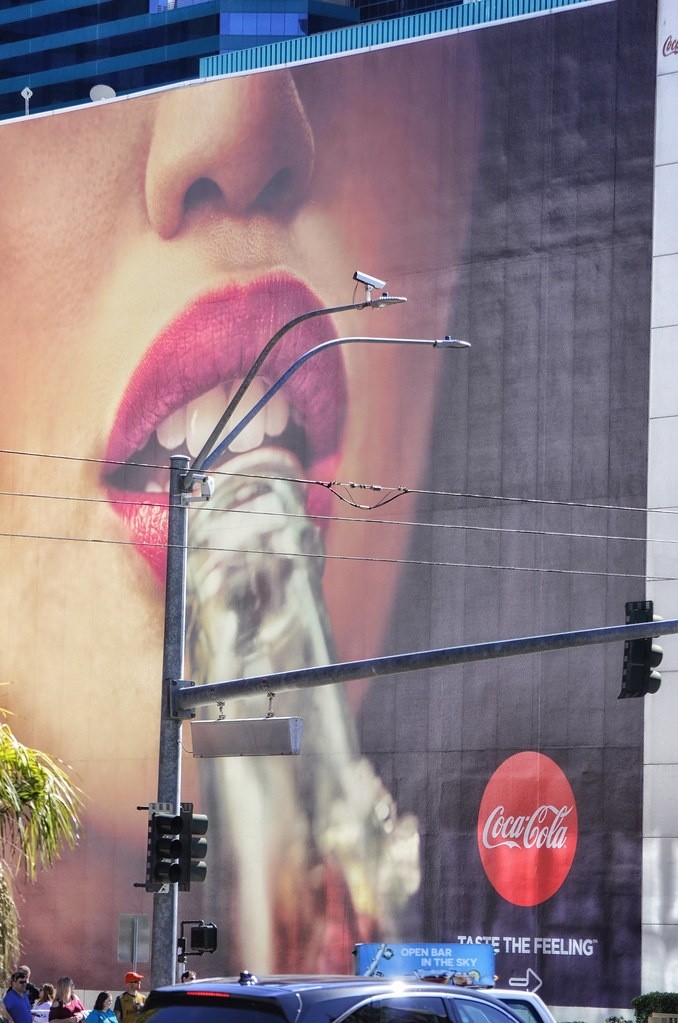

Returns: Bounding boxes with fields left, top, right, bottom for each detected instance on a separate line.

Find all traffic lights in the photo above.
left=617, top=600, right=663, bottom=699
left=191, top=923, right=218, bottom=952
left=146, top=803, right=183, bottom=894
left=178, top=803, right=208, bottom=892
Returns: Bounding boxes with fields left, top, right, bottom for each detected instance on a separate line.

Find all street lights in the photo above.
left=149, top=295, right=476, bottom=992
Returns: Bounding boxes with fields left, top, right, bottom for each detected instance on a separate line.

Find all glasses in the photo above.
left=188, top=978, right=196, bottom=980
left=40, top=989, right=44, bottom=992
left=14, top=980, right=27, bottom=984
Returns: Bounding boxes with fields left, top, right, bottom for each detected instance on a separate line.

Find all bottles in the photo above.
left=178, top=447, right=405, bottom=978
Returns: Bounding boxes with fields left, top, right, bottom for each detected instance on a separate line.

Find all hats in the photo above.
left=125, top=972, right=144, bottom=983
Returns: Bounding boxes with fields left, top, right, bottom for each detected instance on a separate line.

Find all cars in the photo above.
left=133, top=971, right=527, bottom=1023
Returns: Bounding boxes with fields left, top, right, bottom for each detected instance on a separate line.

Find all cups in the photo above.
left=413, top=968, right=456, bottom=984
left=453, top=973, right=475, bottom=986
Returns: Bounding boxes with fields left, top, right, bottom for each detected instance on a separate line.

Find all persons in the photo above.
left=7, top=962, right=201, bottom=1023
